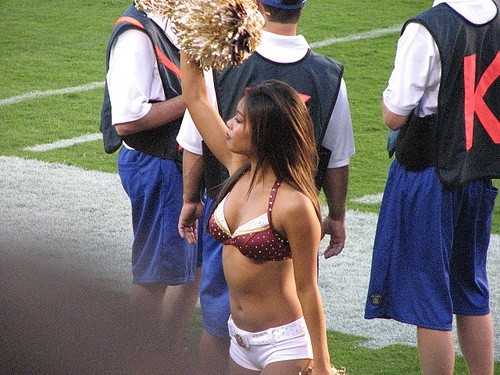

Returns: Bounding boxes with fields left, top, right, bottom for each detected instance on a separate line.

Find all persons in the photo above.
left=178, top=48, right=335, bottom=375
left=100, top=0, right=203, bottom=329
left=176, top=0, right=355, bottom=375
left=365, top=0, right=500, bottom=374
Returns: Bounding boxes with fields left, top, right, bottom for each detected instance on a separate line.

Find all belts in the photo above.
left=227, top=318, right=308, bottom=348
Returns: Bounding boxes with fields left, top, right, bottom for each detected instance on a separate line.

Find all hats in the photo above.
left=259, top=0, right=307, bottom=9
